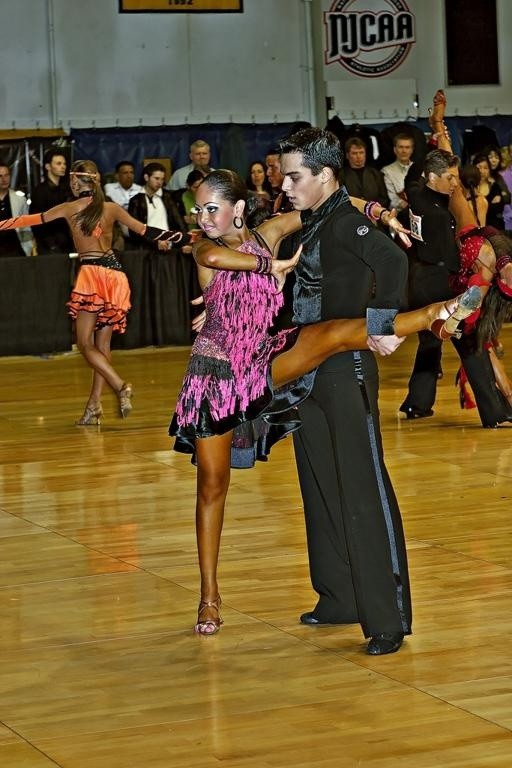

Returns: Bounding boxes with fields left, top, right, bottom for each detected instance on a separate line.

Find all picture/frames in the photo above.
left=118, top=0, right=243, bottom=14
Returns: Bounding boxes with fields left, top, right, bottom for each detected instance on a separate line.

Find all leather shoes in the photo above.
left=368, top=633, right=405, bottom=655
left=300, top=611, right=324, bottom=625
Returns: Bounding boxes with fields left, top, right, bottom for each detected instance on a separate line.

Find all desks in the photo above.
left=0, top=239, right=300, bottom=356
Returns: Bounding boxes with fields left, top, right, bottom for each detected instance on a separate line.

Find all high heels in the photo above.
left=195, top=593, right=223, bottom=635
left=76, top=405, right=104, bottom=425
left=406, top=406, right=434, bottom=419
left=428, top=90, right=447, bottom=129
left=430, top=286, right=484, bottom=342
left=118, top=381, right=133, bottom=419
left=489, top=418, right=512, bottom=431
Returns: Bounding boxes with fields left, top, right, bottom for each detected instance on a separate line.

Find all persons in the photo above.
left=168, top=168, right=482, bottom=635
left=190, top=128, right=413, bottom=656
left=428, top=88, right=511, bottom=409
left=0, top=132, right=512, bottom=256
left=1, top=160, right=199, bottom=426
left=399, top=149, right=511, bottom=428
